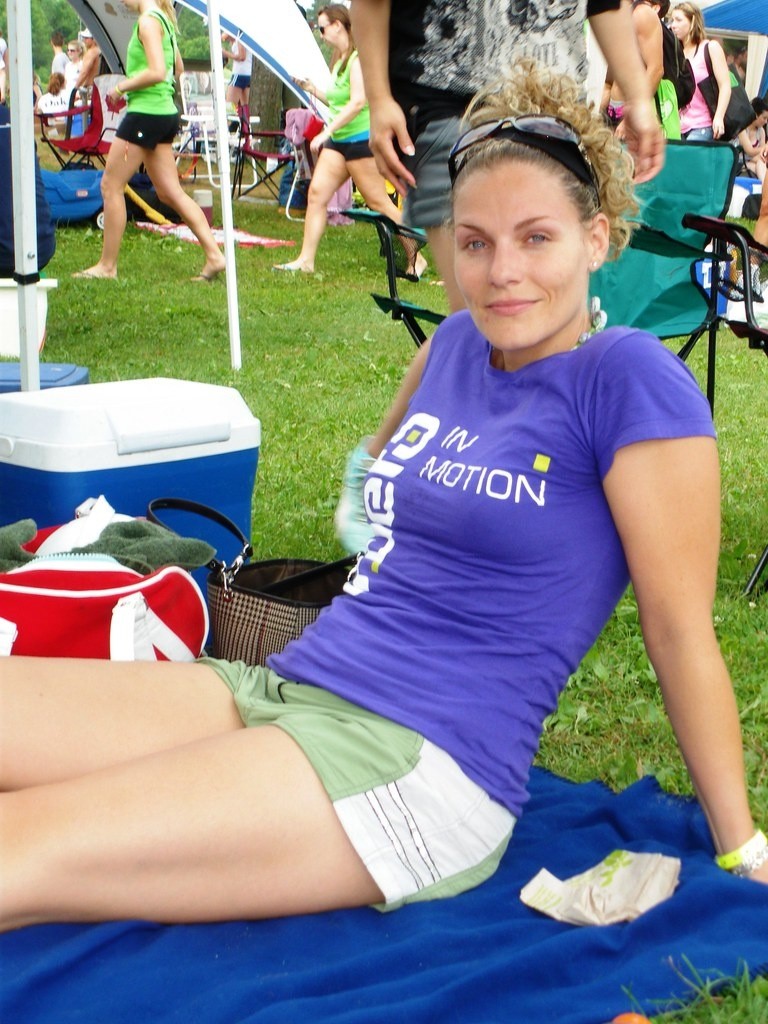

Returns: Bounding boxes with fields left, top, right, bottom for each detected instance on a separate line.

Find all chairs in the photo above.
left=39, top=74, right=134, bottom=170
left=230, top=107, right=318, bottom=203
left=338, top=142, right=767, bottom=427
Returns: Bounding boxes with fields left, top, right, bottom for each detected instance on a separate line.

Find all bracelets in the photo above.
left=733, top=853, right=767, bottom=879
left=115, top=83, right=125, bottom=96
left=713, top=827, right=767, bottom=869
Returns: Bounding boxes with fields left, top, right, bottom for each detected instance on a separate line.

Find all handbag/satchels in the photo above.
left=697, top=41, right=757, bottom=140
left=653, top=78, right=682, bottom=141
left=0, top=516, right=210, bottom=662
left=147, top=498, right=364, bottom=669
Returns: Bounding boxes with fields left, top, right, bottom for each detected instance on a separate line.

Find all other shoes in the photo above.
left=333, top=438, right=373, bottom=556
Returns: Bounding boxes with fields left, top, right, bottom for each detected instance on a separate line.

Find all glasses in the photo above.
left=67, top=49, right=78, bottom=52
left=320, top=22, right=335, bottom=35
left=81, top=28, right=93, bottom=38
left=447, top=113, right=603, bottom=216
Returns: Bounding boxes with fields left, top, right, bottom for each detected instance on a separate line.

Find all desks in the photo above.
left=174, top=114, right=261, bottom=188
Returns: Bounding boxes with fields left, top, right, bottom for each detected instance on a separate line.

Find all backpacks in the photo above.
left=663, top=24, right=695, bottom=109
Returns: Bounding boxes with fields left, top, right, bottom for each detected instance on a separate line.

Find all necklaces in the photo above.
left=573, top=296, right=607, bottom=353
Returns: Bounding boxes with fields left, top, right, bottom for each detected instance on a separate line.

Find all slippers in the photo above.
left=71, top=268, right=118, bottom=279
left=271, top=264, right=314, bottom=275
left=190, top=272, right=226, bottom=283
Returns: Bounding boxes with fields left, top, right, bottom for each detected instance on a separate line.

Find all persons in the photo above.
left=0, top=0, right=768, bottom=284
left=1, top=70, right=768, bottom=930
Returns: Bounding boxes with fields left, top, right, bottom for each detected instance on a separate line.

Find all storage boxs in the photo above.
left=0, top=276, right=59, bottom=359
left=0, top=377, right=263, bottom=649
left=0, top=362, right=91, bottom=395
